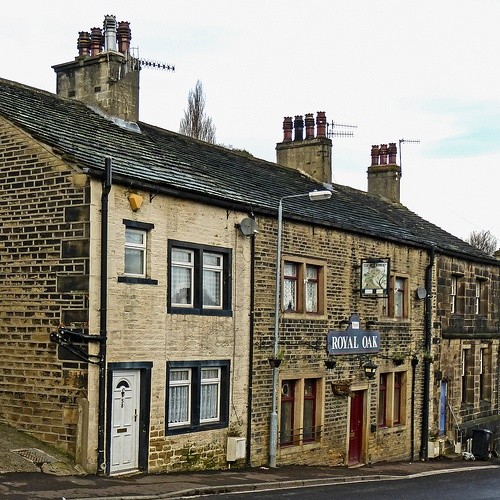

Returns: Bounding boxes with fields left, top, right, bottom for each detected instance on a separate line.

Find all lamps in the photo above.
left=365, top=361, right=377, bottom=379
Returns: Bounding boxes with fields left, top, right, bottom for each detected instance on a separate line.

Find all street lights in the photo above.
left=269, top=190, right=334, bottom=469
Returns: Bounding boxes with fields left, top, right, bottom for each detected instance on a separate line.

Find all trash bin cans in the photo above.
left=471, top=429, right=494, bottom=461
